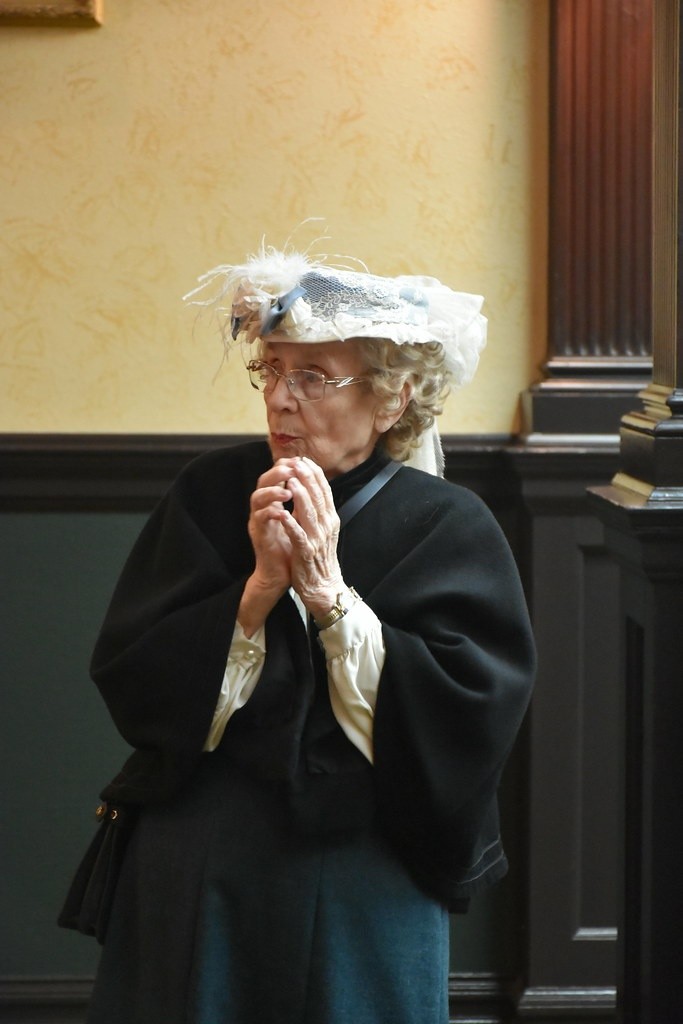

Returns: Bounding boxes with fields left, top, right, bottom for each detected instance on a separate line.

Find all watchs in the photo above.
left=314, top=586, right=363, bottom=632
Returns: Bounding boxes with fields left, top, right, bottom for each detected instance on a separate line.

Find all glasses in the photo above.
left=246, top=359, right=371, bottom=403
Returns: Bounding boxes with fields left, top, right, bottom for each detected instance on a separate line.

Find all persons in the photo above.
left=56, top=245, right=538, bottom=1024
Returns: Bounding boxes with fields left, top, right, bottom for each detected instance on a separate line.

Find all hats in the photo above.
left=182, top=216, right=487, bottom=478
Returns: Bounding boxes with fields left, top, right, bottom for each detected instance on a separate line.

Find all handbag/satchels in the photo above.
left=58, top=801, right=125, bottom=937
